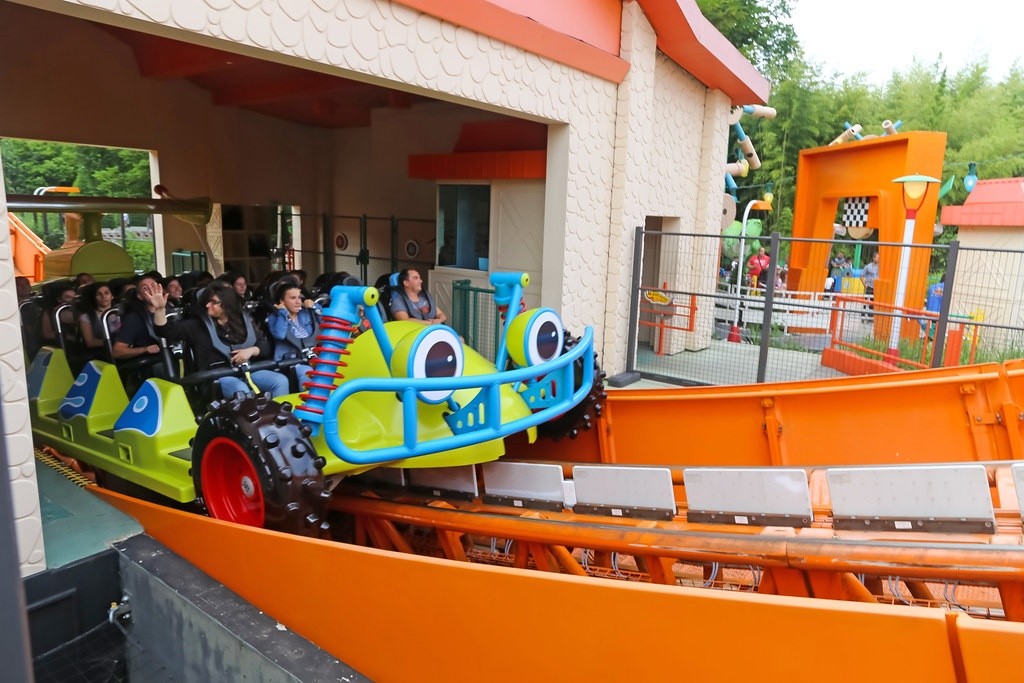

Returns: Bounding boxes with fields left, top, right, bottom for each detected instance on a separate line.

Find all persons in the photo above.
left=726, top=247, right=880, bottom=322
left=12, top=269, right=446, bottom=403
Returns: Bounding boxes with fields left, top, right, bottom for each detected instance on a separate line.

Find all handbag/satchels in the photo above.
left=760, top=269, right=768, bottom=284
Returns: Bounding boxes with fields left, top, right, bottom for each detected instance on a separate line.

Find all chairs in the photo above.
left=18, top=271, right=395, bottom=413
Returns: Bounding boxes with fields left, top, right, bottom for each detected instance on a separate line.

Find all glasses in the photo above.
left=207, top=299, right=222, bottom=305
